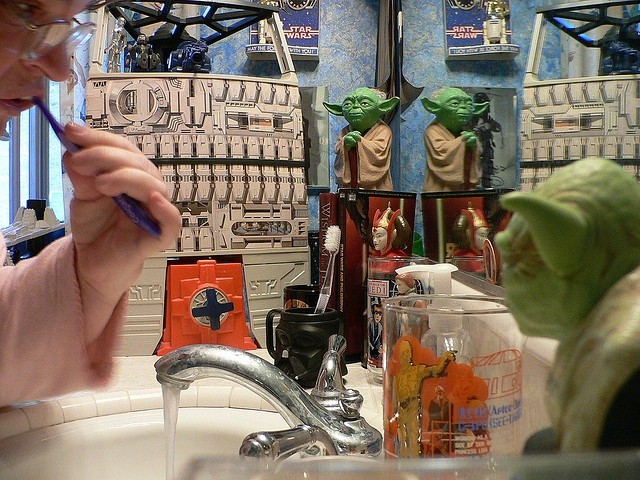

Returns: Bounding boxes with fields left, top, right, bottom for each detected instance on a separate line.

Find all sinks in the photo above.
left=2, top=386, right=389, bottom=480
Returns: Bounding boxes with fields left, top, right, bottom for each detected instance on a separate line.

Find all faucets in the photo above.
left=142, top=330, right=381, bottom=470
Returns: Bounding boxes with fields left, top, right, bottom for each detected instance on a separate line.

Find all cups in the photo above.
left=367, top=257, right=431, bottom=386
left=283, top=285, right=318, bottom=309
left=265, top=307, right=340, bottom=390
left=380, top=293, right=523, bottom=458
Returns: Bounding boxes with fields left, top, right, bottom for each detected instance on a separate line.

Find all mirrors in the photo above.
left=401, top=1, right=640, bottom=296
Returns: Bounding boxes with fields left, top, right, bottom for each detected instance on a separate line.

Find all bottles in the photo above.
left=395, top=263, right=476, bottom=370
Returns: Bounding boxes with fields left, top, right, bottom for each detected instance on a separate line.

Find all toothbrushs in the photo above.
left=36, top=93, right=160, bottom=241
left=311, top=225, right=342, bottom=316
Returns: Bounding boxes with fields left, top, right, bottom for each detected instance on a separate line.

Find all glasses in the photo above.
left=3, top=3, right=97, bottom=61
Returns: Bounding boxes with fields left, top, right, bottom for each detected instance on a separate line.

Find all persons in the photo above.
left=362, top=303, right=384, bottom=370
left=0, top=0, right=182, bottom=410
